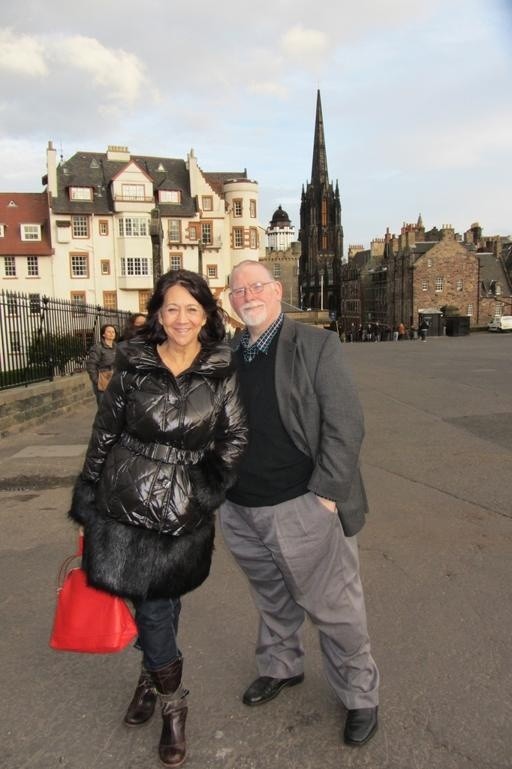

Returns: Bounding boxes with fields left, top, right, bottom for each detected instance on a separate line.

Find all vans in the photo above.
left=488, top=315, right=512, bottom=332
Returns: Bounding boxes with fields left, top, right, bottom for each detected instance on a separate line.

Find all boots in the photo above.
left=140, top=651, right=188, bottom=767
left=123, top=656, right=157, bottom=731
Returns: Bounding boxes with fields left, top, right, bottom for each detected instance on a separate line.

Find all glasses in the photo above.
left=229, top=280, right=274, bottom=299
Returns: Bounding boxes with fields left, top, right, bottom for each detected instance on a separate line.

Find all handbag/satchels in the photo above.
left=95, top=367, right=115, bottom=392
left=46, top=535, right=138, bottom=656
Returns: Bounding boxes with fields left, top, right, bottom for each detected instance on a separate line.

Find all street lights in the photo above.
left=318, top=268, right=325, bottom=311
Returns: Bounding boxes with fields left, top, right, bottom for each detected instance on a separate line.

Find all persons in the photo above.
left=67, top=268, right=251, bottom=769
left=85, top=324, right=120, bottom=406
left=215, top=260, right=381, bottom=748
left=329, top=316, right=432, bottom=344
left=128, top=313, right=149, bottom=329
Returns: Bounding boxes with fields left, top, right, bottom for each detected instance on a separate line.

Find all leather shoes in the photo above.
left=342, top=705, right=380, bottom=747
left=240, top=670, right=305, bottom=710
